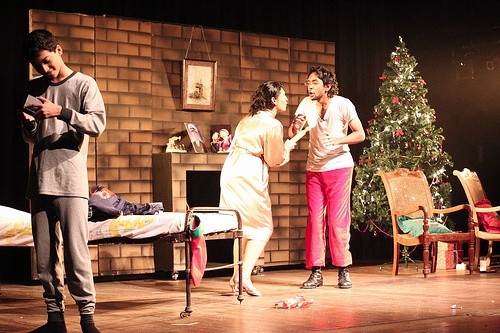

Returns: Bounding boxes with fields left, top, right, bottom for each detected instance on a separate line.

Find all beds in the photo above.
left=0, top=206, right=243, bottom=317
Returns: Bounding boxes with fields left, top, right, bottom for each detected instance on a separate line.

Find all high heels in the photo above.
left=229, top=275, right=262, bottom=296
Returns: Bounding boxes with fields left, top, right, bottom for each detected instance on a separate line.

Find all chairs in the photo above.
left=378, top=167, right=474, bottom=277
left=449, top=168, right=500, bottom=273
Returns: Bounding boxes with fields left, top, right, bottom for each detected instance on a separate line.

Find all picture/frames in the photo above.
left=181, top=59, right=218, bottom=112
left=184, top=122, right=209, bottom=153
left=209, top=125, right=231, bottom=154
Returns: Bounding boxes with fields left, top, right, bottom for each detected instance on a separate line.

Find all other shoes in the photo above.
left=27, top=312, right=68, bottom=333
left=79, top=313, right=103, bottom=333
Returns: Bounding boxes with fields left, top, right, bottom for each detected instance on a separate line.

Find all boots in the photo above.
left=302, top=267, right=324, bottom=289
left=337, top=266, right=353, bottom=289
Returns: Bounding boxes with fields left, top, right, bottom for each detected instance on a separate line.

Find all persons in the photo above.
left=218, top=82, right=311, bottom=297
left=288, top=65, right=366, bottom=289
left=210, top=129, right=231, bottom=153
left=188, top=124, right=206, bottom=152
left=21, top=29, right=107, bottom=333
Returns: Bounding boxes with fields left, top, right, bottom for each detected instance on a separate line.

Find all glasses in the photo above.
left=304, top=81, right=326, bottom=86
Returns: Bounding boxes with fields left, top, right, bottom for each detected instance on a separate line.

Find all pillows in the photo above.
left=475, top=200, right=500, bottom=234
left=396, top=215, right=454, bottom=238
